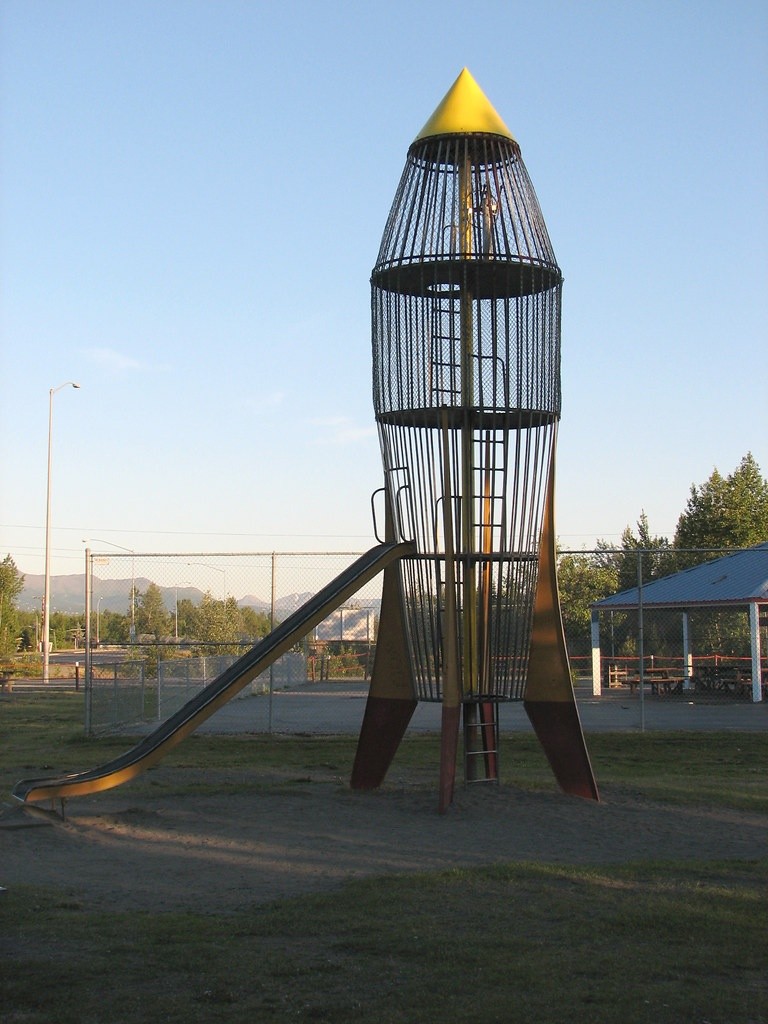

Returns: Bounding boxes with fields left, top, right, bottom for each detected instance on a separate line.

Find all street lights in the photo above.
left=96, top=596, right=103, bottom=645
left=82, top=538, right=136, bottom=661
left=188, top=563, right=227, bottom=612
left=41, top=380, right=81, bottom=690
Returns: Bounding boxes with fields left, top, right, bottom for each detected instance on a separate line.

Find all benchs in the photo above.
left=622, top=667, right=759, bottom=696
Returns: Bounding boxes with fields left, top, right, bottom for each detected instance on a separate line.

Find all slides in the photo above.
left=12, top=538, right=418, bottom=805
